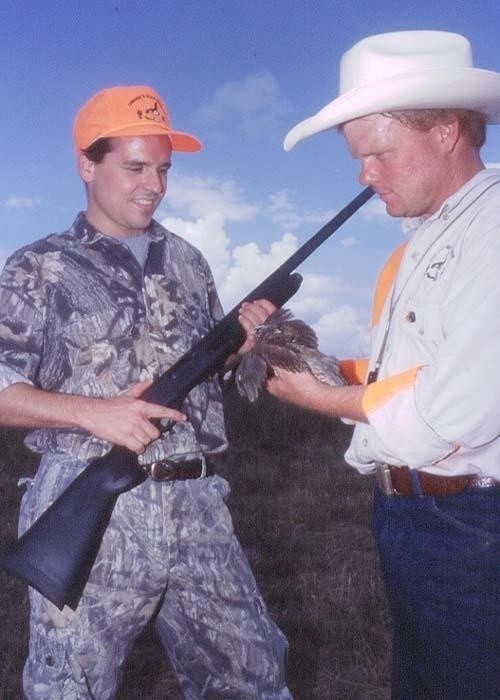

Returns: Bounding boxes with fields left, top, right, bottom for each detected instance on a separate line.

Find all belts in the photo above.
left=377, top=464, right=500, bottom=496
left=142, top=454, right=217, bottom=482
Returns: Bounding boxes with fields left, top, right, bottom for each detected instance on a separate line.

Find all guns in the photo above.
left=0, top=186, right=375, bottom=611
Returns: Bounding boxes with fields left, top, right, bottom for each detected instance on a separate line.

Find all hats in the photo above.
left=283, top=29, right=500, bottom=152
left=72, top=86, right=202, bottom=152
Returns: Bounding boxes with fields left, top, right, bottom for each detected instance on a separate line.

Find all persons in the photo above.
left=1, top=85, right=298, bottom=700
left=262, top=31, right=500, bottom=700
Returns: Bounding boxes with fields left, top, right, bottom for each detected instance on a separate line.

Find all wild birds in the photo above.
left=235, top=307, right=349, bottom=403
